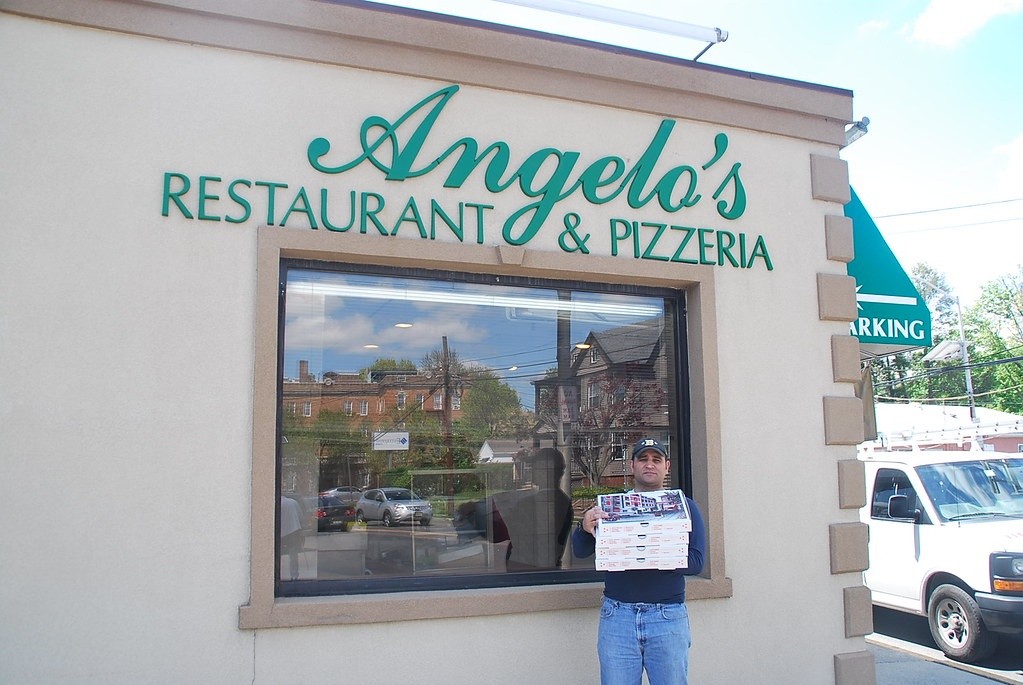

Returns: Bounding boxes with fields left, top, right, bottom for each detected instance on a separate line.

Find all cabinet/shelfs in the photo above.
left=406, top=460, right=514, bottom=571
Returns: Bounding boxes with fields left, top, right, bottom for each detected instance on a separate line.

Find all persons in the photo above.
left=461, top=447, right=575, bottom=573
left=281, top=495, right=302, bottom=580
left=572, top=437, right=706, bottom=685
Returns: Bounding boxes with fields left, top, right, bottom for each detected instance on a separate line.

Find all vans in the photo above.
left=852, top=451, right=1022, bottom=664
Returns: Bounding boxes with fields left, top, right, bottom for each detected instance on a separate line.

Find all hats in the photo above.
left=631, top=438, right=668, bottom=459
left=520, top=447, right=565, bottom=470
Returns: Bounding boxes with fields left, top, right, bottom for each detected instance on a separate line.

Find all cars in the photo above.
left=354, top=487, right=432, bottom=527
left=318, top=485, right=363, bottom=501
left=276, top=494, right=359, bottom=532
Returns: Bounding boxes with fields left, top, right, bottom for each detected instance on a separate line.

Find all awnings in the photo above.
left=844, top=182, right=933, bottom=362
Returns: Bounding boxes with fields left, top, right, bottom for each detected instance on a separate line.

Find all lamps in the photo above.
left=840, top=117, right=874, bottom=151
left=485, top=0, right=730, bottom=63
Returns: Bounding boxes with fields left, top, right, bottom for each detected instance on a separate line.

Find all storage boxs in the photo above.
left=595, top=489, right=692, bottom=569
